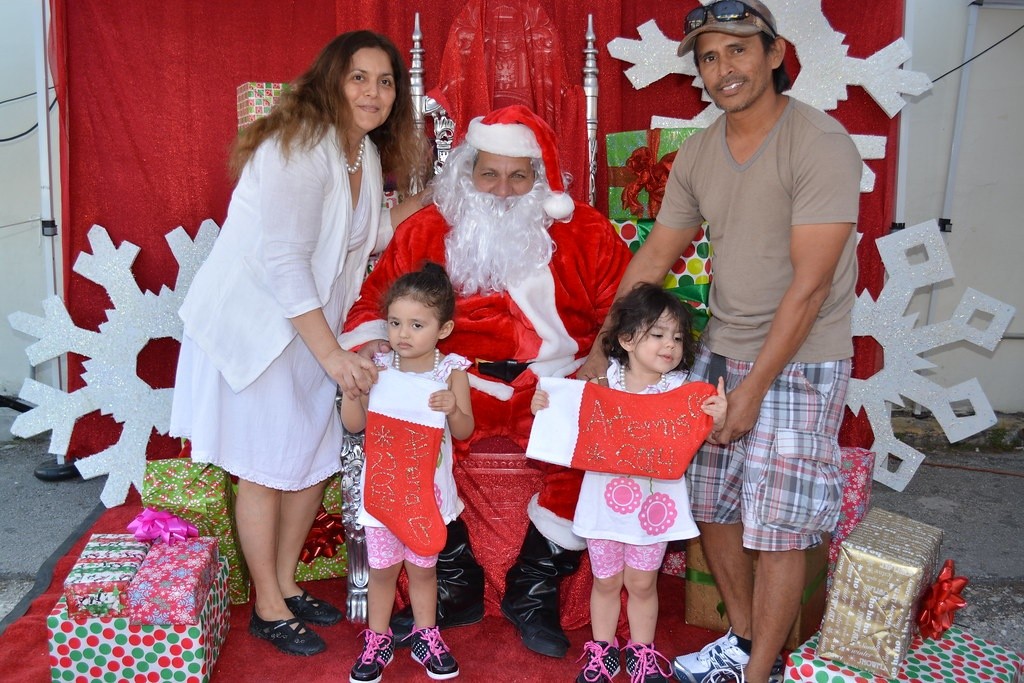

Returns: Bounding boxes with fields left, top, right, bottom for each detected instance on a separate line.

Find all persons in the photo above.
left=530, top=281, right=727, bottom=683
left=338, top=105, right=634, bottom=659
left=170, top=30, right=435, bottom=655
left=577, top=0, right=863, bottom=683
left=341, top=263, right=474, bottom=683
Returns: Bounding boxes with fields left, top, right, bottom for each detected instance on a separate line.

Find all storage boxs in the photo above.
left=606, top=128, right=715, bottom=345
left=685, top=522, right=1024, bottom=683
left=47, top=458, right=344, bottom=683
left=235, top=83, right=300, bottom=139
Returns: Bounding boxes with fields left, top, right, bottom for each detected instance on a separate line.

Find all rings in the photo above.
left=598, top=377, right=606, bottom=379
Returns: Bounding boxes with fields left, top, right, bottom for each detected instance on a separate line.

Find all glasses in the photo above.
left=684, top=0, right=778, bottom=38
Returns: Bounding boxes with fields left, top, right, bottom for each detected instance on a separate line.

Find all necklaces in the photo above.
left=343, top=137, right=365, bottom=174
left=620, top=366, right=666, bottom=392
left=395, top=349, right=439, bottom=381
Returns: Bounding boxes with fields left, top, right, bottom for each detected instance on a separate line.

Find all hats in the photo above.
left=676, top=0, right=776, bottom=57
left=464, top=106, right=574, bottom=220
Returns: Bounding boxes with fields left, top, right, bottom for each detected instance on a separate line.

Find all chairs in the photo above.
left=340, top=10, right=601, bottom=622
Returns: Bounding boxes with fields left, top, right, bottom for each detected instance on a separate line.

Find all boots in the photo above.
left=379, top=515, right=484, bottom=648
left=500, top=493, right=588, bottom=658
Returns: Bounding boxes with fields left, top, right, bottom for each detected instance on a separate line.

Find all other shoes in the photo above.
left=284, top=587, right=342, bottom=627
left=248, top=603, right=326, bottom=657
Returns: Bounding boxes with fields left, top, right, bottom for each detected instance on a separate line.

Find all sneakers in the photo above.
left=401, top=625, right=459, bottom=680
left=348, top=627, right=395, bottom=683
left=668, top=627, right=784, bottom=683
left=574, top=636, right=621, bottom=683
left=619, top=639, right=673, bottom=683
